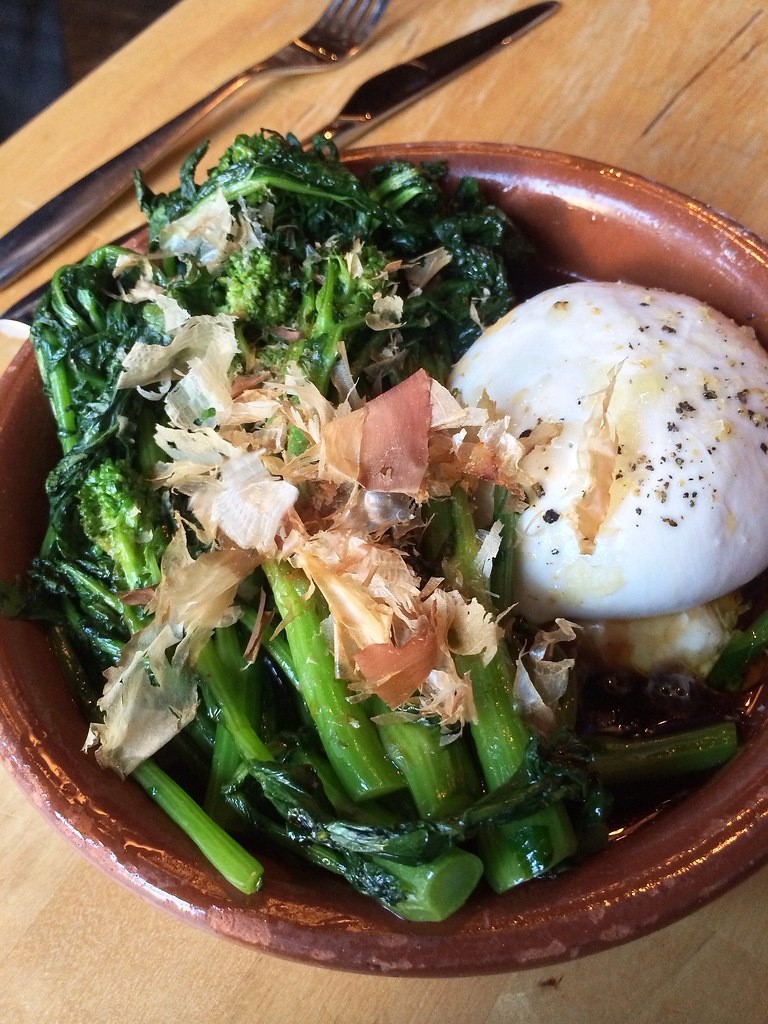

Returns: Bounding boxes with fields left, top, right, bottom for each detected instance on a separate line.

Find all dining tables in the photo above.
left=0, top=0, right=768, bottom=1024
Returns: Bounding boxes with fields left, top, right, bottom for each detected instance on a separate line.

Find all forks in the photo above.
left=0, top=0, right=391, bottom=289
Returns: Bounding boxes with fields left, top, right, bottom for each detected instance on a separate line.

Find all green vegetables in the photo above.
left=0, top=129, right=767, bottom=925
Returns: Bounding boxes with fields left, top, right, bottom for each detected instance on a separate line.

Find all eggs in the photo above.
left=447, top=283, right=767, bottom=620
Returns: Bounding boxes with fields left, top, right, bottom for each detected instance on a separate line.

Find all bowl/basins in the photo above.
left=0, top=139, right=768, bottom=979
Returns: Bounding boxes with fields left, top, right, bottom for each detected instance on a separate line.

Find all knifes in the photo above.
left=0, top=0, right=562, bottom=329
